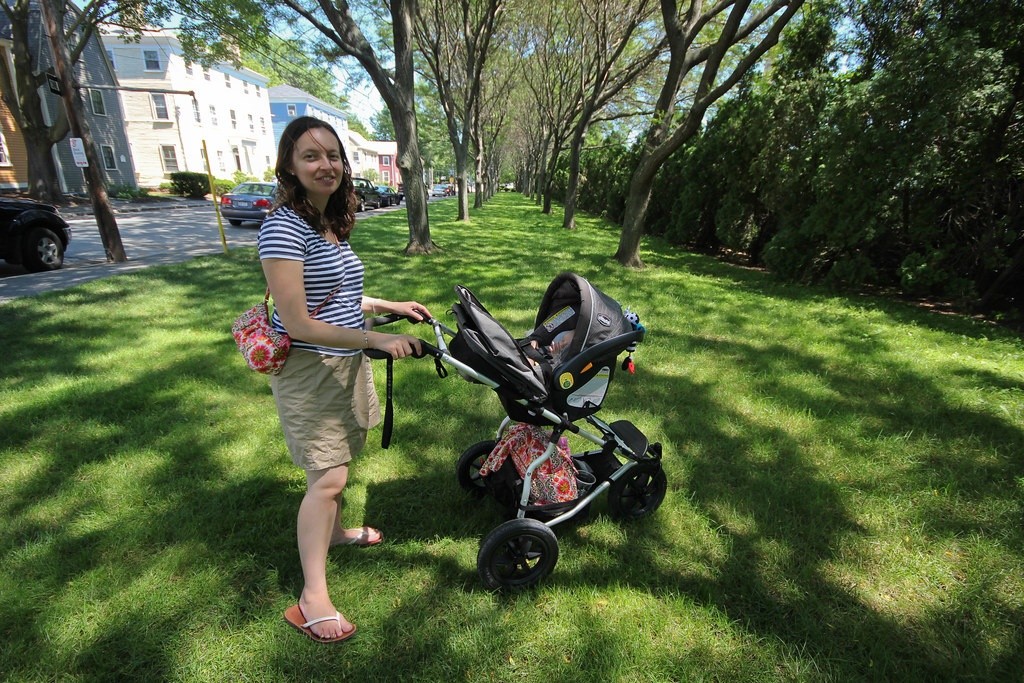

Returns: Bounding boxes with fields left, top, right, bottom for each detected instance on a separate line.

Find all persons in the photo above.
left=257, top=116, right=433, bottom=643
left=531, top=329, right=575, bottom=365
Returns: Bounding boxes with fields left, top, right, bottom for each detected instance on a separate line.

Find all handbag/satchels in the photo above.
left=232, top=302, right=291, bottom=376
left=479, top=423, right=578, bottom=516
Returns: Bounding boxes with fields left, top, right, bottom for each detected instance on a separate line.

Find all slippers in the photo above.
left=330, top=527, right=383, bottom=547
left=284, top=605, right=356, bottom=642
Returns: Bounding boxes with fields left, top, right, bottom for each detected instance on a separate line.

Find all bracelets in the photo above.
left=372, top=298, right=376, bottom=313
left=364, top=329, right=368, bottom=348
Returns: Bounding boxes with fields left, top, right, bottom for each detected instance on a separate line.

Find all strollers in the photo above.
left=363, top=266, right=667, bottom=598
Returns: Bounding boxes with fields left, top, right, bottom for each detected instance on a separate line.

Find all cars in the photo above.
left=350, top=177, right=382, bottom=212
left=0, top=193, right=71, bottom=270
left=432, top=185, right=451, bottom=196
left=219, top=179, right=283, bottom=226
left=375, top=185, right=401, bottom=206
